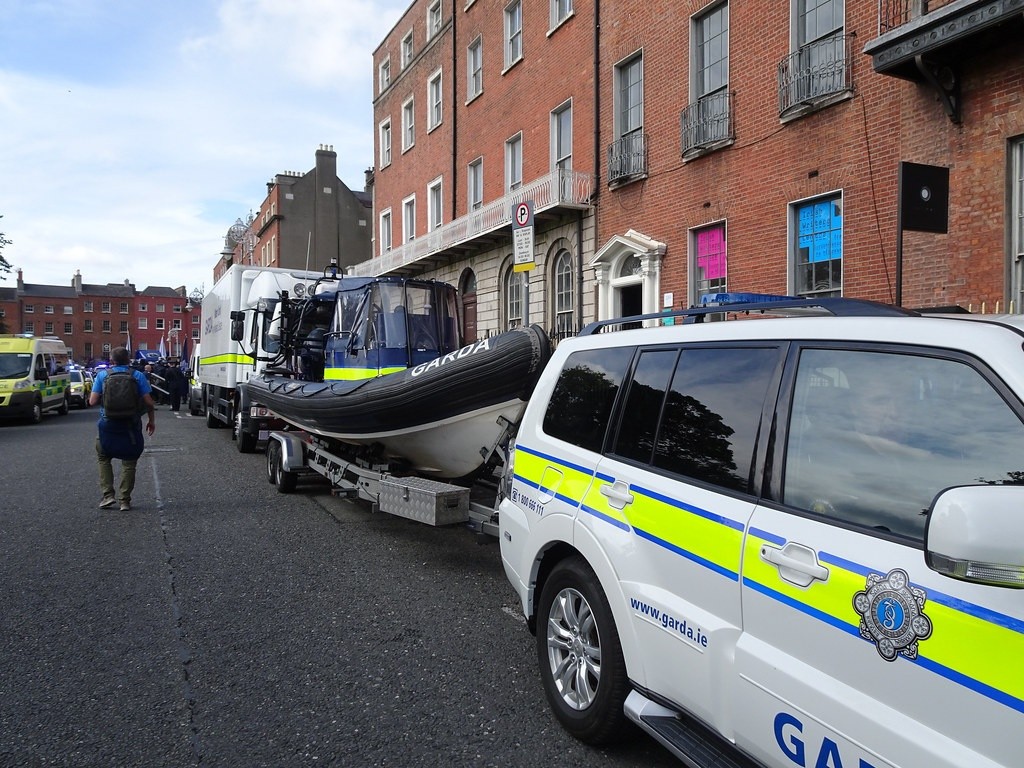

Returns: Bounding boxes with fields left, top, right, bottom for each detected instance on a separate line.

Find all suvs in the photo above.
left=497, top=291, right=1024, bottom=768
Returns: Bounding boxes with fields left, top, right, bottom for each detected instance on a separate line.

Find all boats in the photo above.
left=246, top=229, right=555, bottom=491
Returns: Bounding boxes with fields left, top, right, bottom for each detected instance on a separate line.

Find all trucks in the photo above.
left=199, top=264, right=359, bottom=453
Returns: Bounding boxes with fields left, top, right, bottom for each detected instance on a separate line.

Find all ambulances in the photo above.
left=0, top=333, right=71, bottom=425
left=70, top=362, right=108, bottom=409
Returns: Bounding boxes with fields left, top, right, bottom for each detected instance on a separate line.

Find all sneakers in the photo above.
left=119, top=506, right=130, bottom=511
left=99, top=497, right=116, bottom=508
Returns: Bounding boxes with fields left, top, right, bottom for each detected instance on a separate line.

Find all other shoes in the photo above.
left=153, top=408, right=158, bottom=411
left=169, top=408, right=180, bottom=412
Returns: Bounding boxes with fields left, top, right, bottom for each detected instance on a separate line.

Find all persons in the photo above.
left=88, top=347, right=156, bottom=510
left=133, top=359, right=190, bottom=412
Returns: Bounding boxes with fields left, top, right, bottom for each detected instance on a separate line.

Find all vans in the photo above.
left=186, top=343, right=205, bottom=416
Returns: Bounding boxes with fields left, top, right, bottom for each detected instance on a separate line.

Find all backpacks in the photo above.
left=102, top=367, right=148, bottom=419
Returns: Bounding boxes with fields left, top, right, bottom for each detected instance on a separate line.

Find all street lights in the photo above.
left=221, top=224, right=255, bottom=266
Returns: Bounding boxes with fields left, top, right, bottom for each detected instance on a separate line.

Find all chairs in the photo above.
left=794, top=385, right=862, bottom=524
